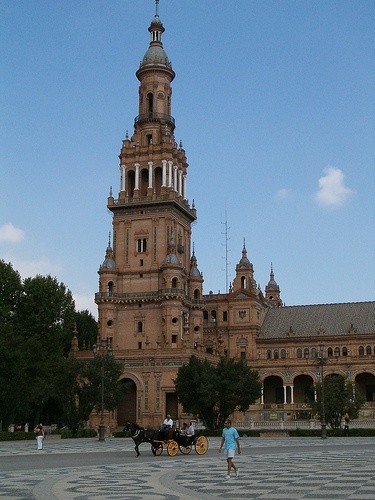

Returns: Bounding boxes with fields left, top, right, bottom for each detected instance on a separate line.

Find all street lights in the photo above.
left=92, top=344, right=114, bottom=442
left=317, top=356, right=327, bottom=440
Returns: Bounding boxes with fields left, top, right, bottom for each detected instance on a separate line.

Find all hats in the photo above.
left=167, top=414, right=171, bottom=417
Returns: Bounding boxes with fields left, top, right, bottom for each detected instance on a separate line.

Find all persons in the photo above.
left=34, top=425, right=44, bottom=449
left=163, top=414, right=173, bottom=428
left=218, top=419, right=241, bottom=480
left=181, top=423, right=187, bottom=430
left=185, top=422, right=195, bottom=435
left=344, top=417, right=349, bottom=429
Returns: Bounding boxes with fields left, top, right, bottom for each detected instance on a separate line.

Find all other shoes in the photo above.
left=235, top=468, right=239, bottom=477
left=224, top=475, right=230, bottom=479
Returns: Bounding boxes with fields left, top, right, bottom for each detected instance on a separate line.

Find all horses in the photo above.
left=121, top=420, right=160, bottom=456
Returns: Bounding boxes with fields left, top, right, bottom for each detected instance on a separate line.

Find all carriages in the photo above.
left=123, top=418, right=209, bottom=457
